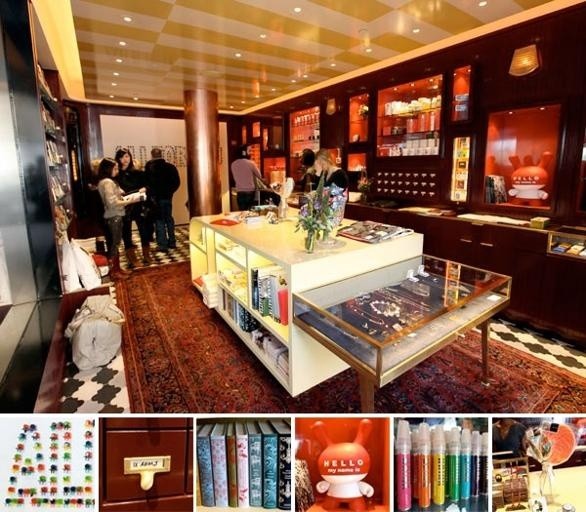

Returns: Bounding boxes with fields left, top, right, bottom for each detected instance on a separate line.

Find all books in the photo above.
left=337, top=220, right=414, bottom=243
left=486, top=174, right=507, bottom=203
left=191, top=227, right=289, bottom=385
left=195, top=419, right=291, bottom=510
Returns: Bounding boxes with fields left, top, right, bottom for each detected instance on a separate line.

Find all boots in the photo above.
left=141, top=245, right=153, bottom=264
left=124, top=244, right=145, bottom=268
left=108, top=251, right=133, bottom=282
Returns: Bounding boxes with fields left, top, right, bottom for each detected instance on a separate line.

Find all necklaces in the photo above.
left=347, top=286, right=432, bottom=325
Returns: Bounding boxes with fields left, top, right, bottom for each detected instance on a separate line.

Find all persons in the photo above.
left=492, top=418, right=531, bottom=473
left=230, top=144, right=262, bottom=210
left=87, top=147, right=180, bottom=280
left=293, top=148, right=316, bottom=192
left=311, top=148, right=349, bottom=198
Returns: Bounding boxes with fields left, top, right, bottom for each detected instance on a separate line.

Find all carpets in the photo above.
left=113, top=254, right=586, bottom=414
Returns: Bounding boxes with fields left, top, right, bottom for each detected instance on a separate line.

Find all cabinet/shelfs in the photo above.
left=34, top=65, right=75, bottom=291
left=224, top=3, right=586, bottom=349
left=291, top=251, right=517, bottom=414
left=183, top=206, right=425, bottom=400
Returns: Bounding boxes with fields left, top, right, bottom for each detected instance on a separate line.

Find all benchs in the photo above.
left=33, top=283, right=133, bottom=413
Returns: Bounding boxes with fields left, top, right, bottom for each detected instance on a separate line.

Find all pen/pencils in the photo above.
left=395, top=419, right=489, bottom=512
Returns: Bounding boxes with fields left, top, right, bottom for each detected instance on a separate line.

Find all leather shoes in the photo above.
left=151, top=246, right=168, bottom=252
